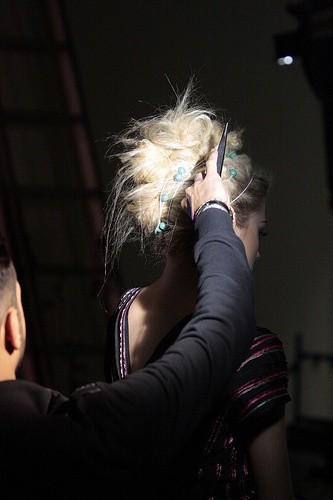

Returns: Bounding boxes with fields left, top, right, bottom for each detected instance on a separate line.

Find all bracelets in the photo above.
left=195, top=199, right=236, bottom=228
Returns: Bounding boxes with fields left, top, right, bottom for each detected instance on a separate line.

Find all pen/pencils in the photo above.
left=217, top=122, right=228, bottom=176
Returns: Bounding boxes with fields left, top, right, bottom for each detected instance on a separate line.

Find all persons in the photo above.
left=104, top=80, right=291, bottom=500
left=0, top=145, right=255, bottom=500
